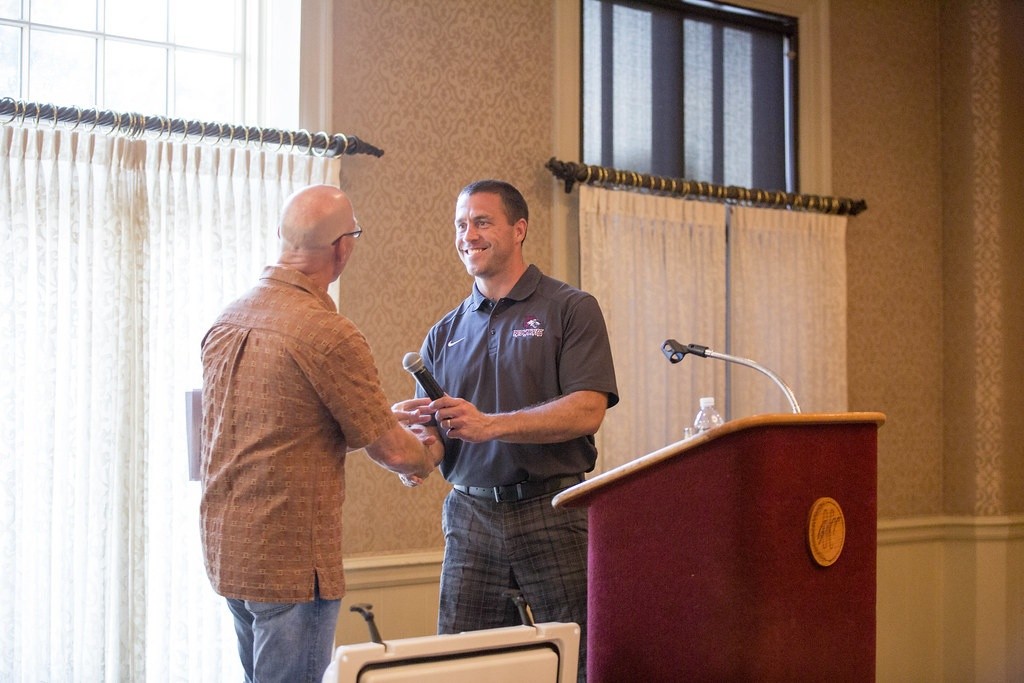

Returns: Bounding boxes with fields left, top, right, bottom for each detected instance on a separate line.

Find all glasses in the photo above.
left=331, top=224, right=363, bottom=246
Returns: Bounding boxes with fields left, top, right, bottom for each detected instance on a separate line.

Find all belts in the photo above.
left=453, top=472, right=586, bottom=504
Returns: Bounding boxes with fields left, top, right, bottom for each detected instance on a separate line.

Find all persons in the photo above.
left=401, top=180, right=620, bottom=683
left=198, top=184, right=437, bottom=683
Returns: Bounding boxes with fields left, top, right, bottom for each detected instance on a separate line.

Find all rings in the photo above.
left=447, top=420, right=452, bottom=429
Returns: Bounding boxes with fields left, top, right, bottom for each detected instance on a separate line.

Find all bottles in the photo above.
left=693, top=397, right=724, bottom=435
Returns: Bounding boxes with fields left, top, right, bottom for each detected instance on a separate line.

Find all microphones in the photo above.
left=402, top=352, right=445, bottom=401
